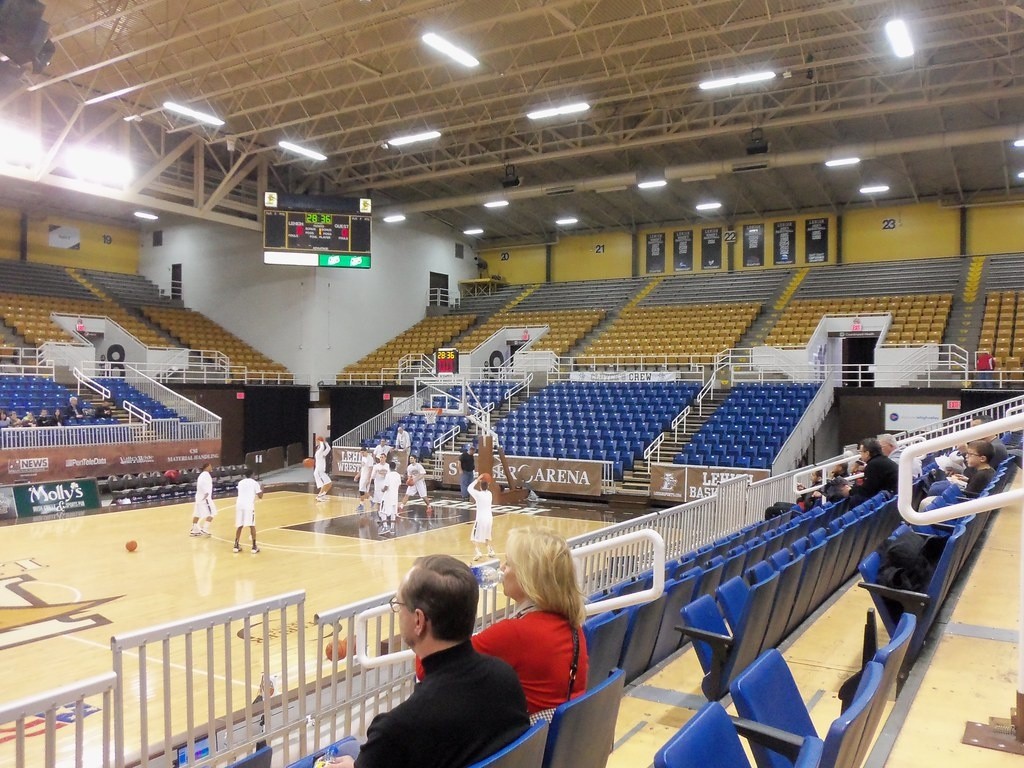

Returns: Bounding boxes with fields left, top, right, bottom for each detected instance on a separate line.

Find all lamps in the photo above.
left=745, top=127, right=771, bottom=155
left=501, top=164, right=520, bottom=190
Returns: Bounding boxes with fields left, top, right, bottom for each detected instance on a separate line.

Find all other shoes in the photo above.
left=473, top=553, right=482, bottom=561
left=488, top=551, right=496, bottom=556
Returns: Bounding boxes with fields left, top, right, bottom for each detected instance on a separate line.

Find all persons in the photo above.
left=467, top=474, right=495, bottom=561
left=765, top=415, right=1008, bottom=522
left=313, top=438, right=332, bottom=502
left=398, top=454, right=433, bottom=513
left=416, top=525, right=589, bottom=715
left=0, top=396, right=112, bottom=428
left=233, top=469, right=264, bottom=553
left=394, top=427, right=411, bottom=466
left=323, top=554, right=530, bottom=768
left=354, top=437, right=401, bottom=534
left=99, top=355, right=106, bottom=376
left=977, top=352, right=995, bottom=389
left=458, top=446, right=476, bottom=501
left=190, top=461, right=216, bottom=537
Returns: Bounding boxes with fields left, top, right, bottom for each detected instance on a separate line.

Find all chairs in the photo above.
left=0, top=263, right=1024, bottom=768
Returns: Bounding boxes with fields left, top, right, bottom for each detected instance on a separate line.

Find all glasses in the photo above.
left=856, top=449, right=868, bottom=454
left=966, top=452, right=980, bottom=457
left=388, top=596, right=407, bottom=611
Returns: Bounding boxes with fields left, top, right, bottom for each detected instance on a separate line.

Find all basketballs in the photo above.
left=302, top=458, right=315, bottom=468
left=344, top=635, right=357, bottom=655
left=406, top=479, right=413, bottom=486
left=126, top=540, right=137, bottom=551
left=325, top=639, right=346, bottom=660
left=317, top=436, right=324, bottom=442
left=481, top=472, right=491, bottom=483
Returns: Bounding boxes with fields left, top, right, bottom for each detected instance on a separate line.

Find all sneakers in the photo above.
left=190, top=530, right=201, bottom=536
left=356, top=505, right=364, bottom=511
left=315, top=495, right=330, bottom=502
left=397, top=507, right=404, bottom=512
left=379, top=527, right=390, bottom=534
left=252, top=547, right=259, bottom=553
left=201, top=527, right=211, bottom=536
left=390, top=528, right=395, bottom=533
left=233, top=545, right=242, bottom=552
left=370, top=500, right=375, bottom=508
left=426, top=507, right=432, bottom=512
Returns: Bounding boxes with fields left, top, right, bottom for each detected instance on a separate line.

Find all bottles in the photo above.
left=312, top=745, right=338, bottom=768
left=470, top=566, right=504, bottom=591
